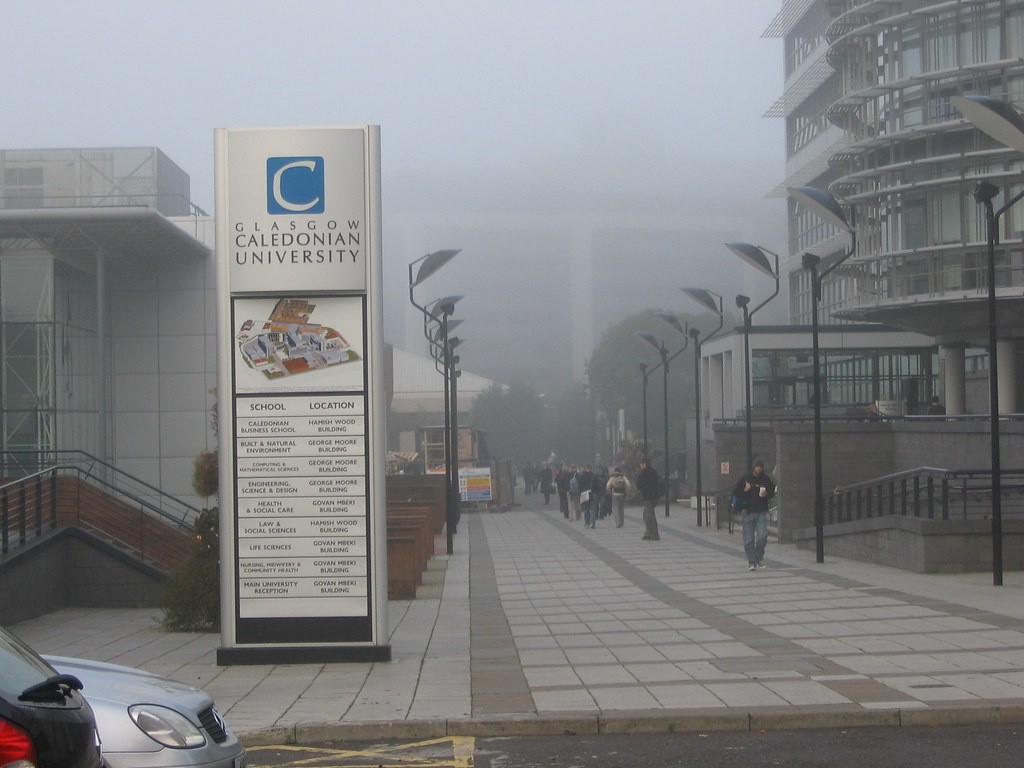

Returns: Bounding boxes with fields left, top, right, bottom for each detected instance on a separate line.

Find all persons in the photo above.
left=928, top=397, right=946, bottom=421
left=734, top=460, right=774, bottom=570
left=524, top=462, right=631, bottom=528
left=637, top=459, right=660, bottom=540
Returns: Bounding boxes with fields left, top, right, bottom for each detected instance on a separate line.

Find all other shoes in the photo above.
left=756, top=561, right=766, bottom=569
left=749, top=561, right=756, bottom=571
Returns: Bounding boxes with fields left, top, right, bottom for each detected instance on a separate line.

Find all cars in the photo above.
left=38, top=654, right=248, bottom=768
left=0, top=626, right=114, bottom=768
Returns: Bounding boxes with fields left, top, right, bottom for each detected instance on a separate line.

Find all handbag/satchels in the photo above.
left=580, top=491, right=589, bottom=504
left=732, top=473, right=746, bottom=512
left=549, top=485, right=556, bottom=494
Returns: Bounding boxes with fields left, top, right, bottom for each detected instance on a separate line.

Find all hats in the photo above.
left=752, top=458, right=764, bottom=469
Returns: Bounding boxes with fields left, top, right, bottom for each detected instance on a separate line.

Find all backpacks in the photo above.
left=646, top=470, right=665, bottom=497
left=612, top=475, right=625, bottom=493
left=569, top=472, right=579, bottom=495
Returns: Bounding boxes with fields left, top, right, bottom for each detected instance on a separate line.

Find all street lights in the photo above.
left=637, top=332, right=664, bottom=458
left=409, top=248, right=462, bottom=556
left=679, top=287, right=723, bottom=527
left=786, top=185, right=857, bottom=565
left=424, top=294, right=466, bottom=532
left=659, top=314, right=689, bottom=517
left=949, top=95, right=1024, bottom=587
left=724, top=242, right=780, bottom=473
